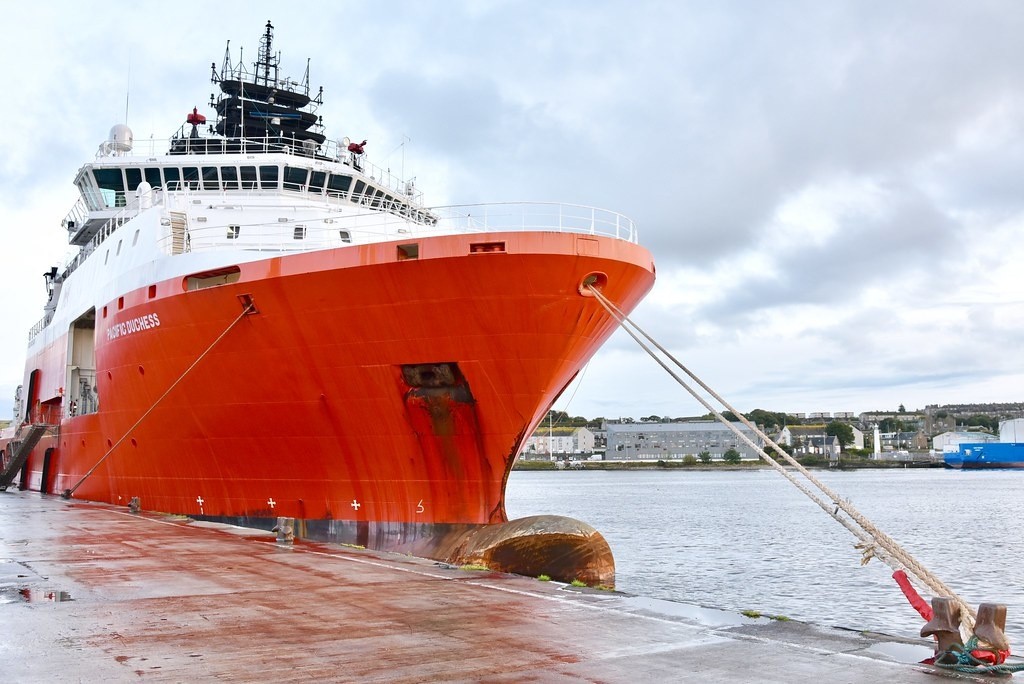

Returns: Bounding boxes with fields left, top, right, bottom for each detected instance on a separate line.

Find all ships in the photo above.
left=12, top=16, right=658, bottom=594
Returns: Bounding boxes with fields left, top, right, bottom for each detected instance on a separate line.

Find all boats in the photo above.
left=942, top=442, right=1024, bottom=469
